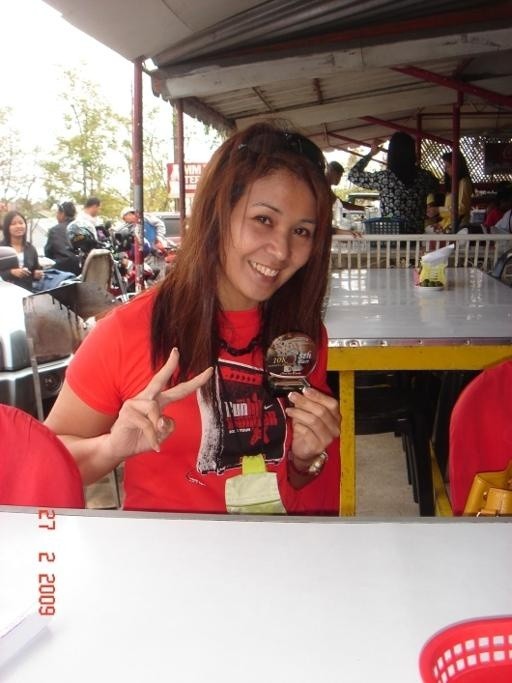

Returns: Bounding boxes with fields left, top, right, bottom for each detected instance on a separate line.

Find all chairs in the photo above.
left=0, top=404, right=85, bottom=509
left=448, top=360, right=512, bottom=516
left=491, top=249, right=512, bottom=279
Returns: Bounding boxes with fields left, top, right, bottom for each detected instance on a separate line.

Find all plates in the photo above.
left=414, top=285, right=445, bottom=291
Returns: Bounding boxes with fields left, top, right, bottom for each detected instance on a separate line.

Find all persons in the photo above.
left=484, top=181, right=512, bottom=245
left=0, top=210, right=44, bottom=292
left=43, top=196, right=167, bottom=275
left=347, top=133, right=475, bottom=268
left=42, top=122, right=342, bottom=517
left=326, top=161, right=361, bottom=237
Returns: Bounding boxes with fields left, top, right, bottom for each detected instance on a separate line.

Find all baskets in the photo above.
left=365, top=217, right=405, bottom=247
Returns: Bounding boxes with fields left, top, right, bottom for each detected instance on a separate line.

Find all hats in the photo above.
left=120, top=208, right=135, bottom=218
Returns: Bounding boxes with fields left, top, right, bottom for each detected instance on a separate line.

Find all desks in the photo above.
left=321, top=267, right=512, bottom=517
left=0, top=506, right=512, bottom=683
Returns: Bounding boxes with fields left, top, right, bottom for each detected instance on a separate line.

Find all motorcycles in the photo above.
left=76, top=227, right=179, bottom=300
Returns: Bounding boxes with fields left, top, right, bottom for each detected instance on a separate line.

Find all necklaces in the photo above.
left=217, top=334, right=261, bottom=356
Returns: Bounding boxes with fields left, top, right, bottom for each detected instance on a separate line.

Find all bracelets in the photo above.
left=285, top=447, right=328, bottom=476
